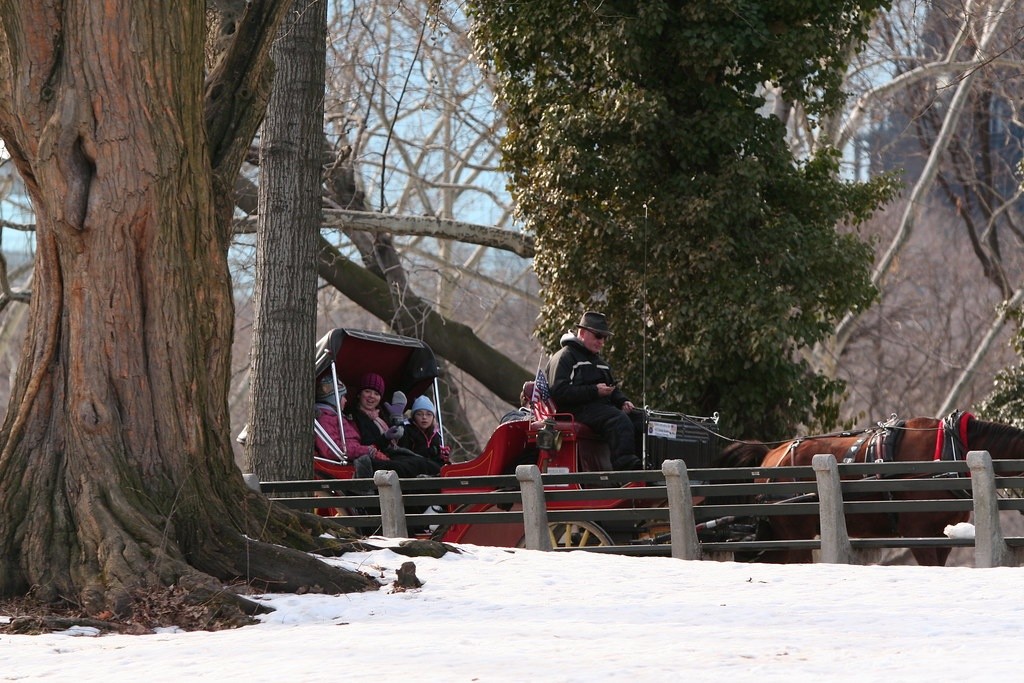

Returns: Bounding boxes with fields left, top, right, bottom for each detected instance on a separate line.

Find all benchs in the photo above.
left=526, top=400, right=602, bottom=443
left=438, top=420, right=535, bottom=513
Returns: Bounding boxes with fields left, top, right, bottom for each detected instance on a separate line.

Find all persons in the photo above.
left=499, top=381, right=534, bottom=425
left=315, top=372, right=448, bottom=511
left=545, top=312, right=646, bottom=471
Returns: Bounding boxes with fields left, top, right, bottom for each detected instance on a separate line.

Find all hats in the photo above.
left=412, top=395, right=435, bottom=416
left=360, top=372, right=385, bottom=396
left=574, top=311, right=615, bottom=336
left=317, top=375, right=346, bottom=405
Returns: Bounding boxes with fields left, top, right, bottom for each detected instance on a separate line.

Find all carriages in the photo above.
left=238, top=326, right=1024, bottom=566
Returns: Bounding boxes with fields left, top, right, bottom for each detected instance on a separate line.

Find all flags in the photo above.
left=532, top=367, right=557, bottom=421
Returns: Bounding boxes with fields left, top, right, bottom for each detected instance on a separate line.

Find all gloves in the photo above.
left=368, top=445, right=389, bottom=462
left=384, top=391, right=407, bottom=418
left=385, top=425, right=404, bottom=439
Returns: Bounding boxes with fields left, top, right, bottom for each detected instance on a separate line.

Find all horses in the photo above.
left=709, top=408, right=1024, bottom=566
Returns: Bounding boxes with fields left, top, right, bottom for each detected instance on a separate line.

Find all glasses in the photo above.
left=585, top=329, right=608, bottom=340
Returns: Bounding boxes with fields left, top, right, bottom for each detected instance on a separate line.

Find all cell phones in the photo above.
left=610, top=381, right=622, bottom=387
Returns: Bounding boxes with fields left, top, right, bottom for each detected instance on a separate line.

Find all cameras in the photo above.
left=392, top=417, right=405, bottom=433
